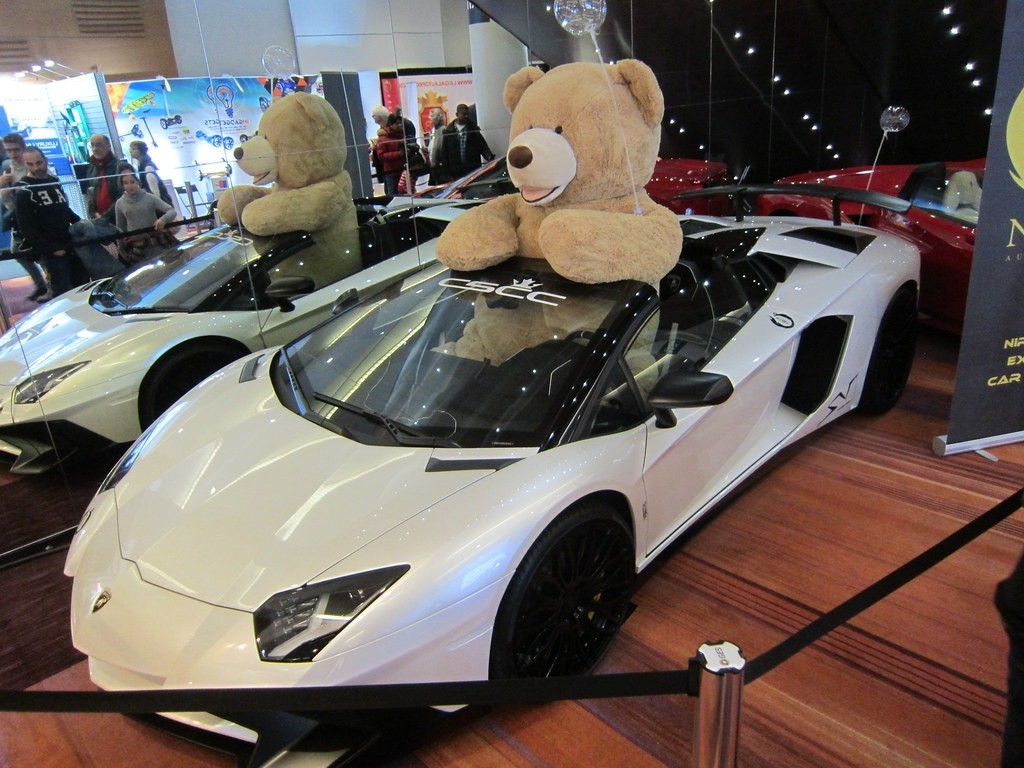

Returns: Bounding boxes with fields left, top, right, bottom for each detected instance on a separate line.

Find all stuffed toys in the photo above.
left=217, top=91, right=362, bottom=304
left=943, top=170, right=981, bottom=214
left=426, top=59, right=684, bottom=420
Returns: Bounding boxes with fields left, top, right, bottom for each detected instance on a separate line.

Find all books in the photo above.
left=415, top=174, right=430, bottom=193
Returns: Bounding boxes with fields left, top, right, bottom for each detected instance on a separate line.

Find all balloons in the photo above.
left=880, top=105, right=910, bottom=131
left=553, top=0, right=607, bottom=37
left=262, top=43, right=296, bottom=80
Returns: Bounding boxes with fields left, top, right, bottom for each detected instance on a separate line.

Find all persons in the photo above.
left=84, top=134, right=130, bottom=268
left=398, top=149, right=429, bottom=194
left=10, top=146, right=85, bottom=301
left=375, top=113, right=413, bottom=197
left=126, top=141, right=181, bottom=245
left=114, top=162, right=178, bottom=266
left=440, top=103, right=496, bottom=184
left=428, top=108, right=449, bottom=186
left=371, top=105, right=393, bottom=197
left=0, top=132, right=56, bottom=303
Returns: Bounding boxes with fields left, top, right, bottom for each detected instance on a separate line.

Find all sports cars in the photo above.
left=757, top=156, right=987, bottom=335
left=411, top=151, right=737, bottom=220
left=1, top=194, right=492, bottom=480
left=60, top=183, right=921, bottom=765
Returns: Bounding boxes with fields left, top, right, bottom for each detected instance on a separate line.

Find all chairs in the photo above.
left=652, top=299, right=682, bottom=376
left=174, top=184, right=214, bottom=229
